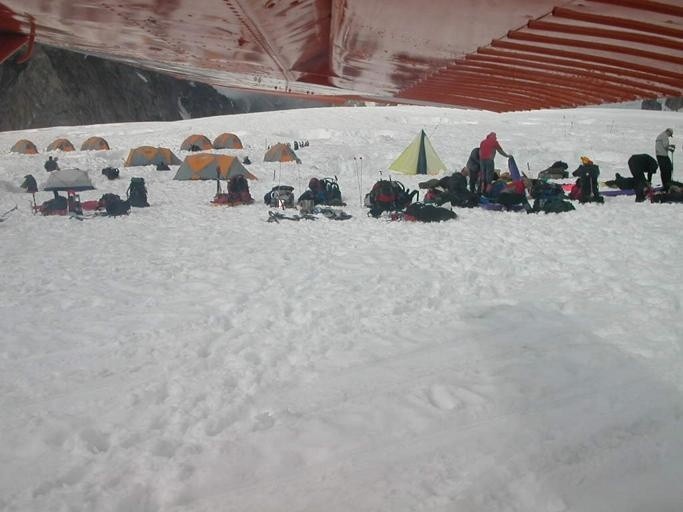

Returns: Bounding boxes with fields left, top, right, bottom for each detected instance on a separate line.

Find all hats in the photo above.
left=667, top=128, right=673, bottom=138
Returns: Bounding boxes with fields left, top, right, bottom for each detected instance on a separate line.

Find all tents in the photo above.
left=8, top=132, right=301, bottom=191
left=386, top=128, right=448, bottom=176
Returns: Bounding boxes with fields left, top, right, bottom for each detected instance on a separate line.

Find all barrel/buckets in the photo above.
left=269, top=186, right=294, bottom=208
left=300, top=199, right=315, bottom=211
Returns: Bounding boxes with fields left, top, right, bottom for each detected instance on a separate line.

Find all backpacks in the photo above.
left=102, top=194, right=130, bottom=217
left=101, top=166, right=120, bottom=181
left=227, top=174, right=254, bottom=207
left=127, top=176, right=150, bottom=207
left=364, top=159, right=576, bottom=223
left=263, top=175, right=346, bottom=210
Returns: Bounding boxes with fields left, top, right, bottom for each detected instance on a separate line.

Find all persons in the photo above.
left=466, top=141, right=480, bottom=195
left=627, top=153, right=660, bottom=202
left=654, top=128, right=675, bottom=192
left=447, top=167, right=477, bottom=208
left=478, top=131, right=512, bottom=196
